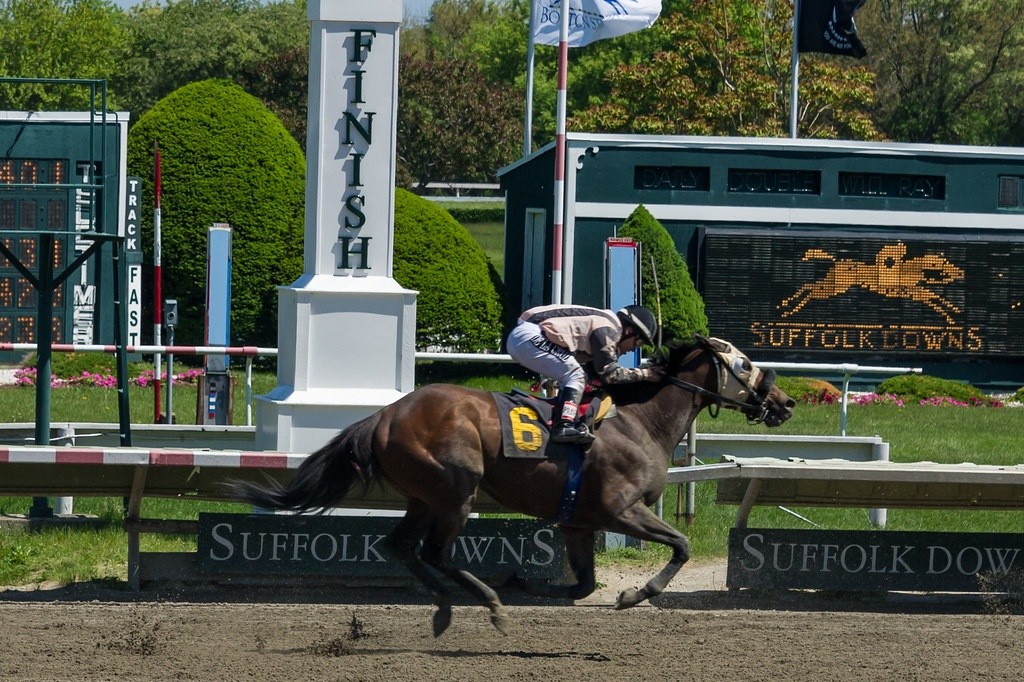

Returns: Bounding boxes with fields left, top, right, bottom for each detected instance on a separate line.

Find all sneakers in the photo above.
left=551, top=419, right=596, bottom=442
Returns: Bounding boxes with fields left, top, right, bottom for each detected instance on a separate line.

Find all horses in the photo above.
left=208, top=333, right=797, bottom=638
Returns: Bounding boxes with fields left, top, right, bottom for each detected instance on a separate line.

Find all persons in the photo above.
left=505, top=303, right=657, bottom=443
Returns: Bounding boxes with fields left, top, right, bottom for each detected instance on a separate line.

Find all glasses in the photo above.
left=635, top=332, right=644, bottom=346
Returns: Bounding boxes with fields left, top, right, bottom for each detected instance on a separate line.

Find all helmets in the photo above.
left=617, top=305, right=657, bottom=347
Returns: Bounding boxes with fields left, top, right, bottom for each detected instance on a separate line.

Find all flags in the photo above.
left=795, top=0, right=869, bottom=60
left=530, top=1, right=662, bottom=49
